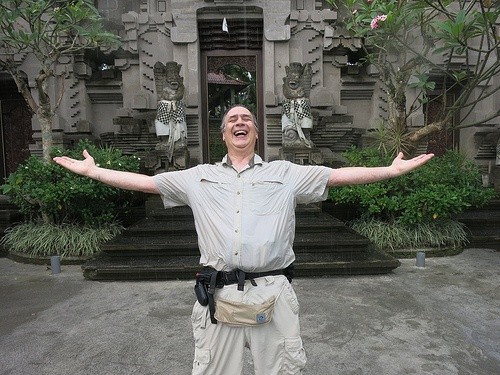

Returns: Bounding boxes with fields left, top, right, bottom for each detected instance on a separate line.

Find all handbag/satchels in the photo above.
left=211, top=291, right=278, bottom=325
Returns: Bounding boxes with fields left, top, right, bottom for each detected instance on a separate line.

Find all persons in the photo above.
left=53, top=104, right=434, bottom=375
